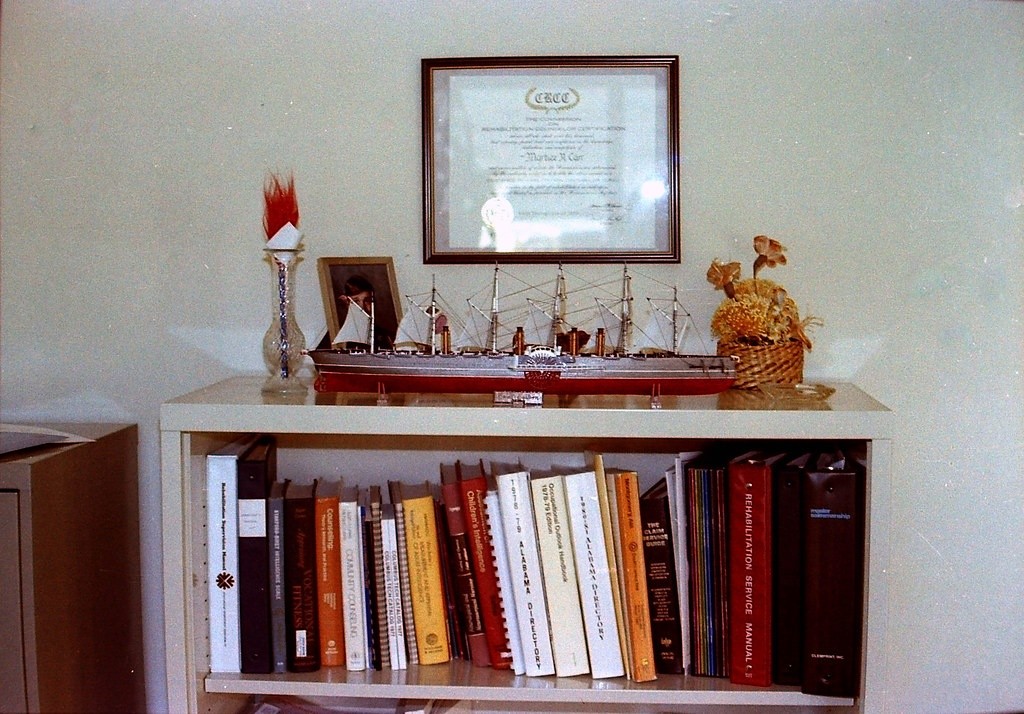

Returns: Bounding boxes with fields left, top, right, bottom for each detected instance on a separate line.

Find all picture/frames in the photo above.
left=317, top=258, right=404, bottom=351
left=422, top=56, right=681, bottom=265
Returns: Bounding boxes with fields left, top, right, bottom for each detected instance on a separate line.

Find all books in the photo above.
left=190, top=434, right=872, bottom=714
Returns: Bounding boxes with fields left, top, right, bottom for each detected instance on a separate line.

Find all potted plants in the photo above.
left=715, top=341, right=804, bottom=384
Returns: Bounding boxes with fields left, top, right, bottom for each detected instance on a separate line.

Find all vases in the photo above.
left=263, top=246, right=310, bottom=395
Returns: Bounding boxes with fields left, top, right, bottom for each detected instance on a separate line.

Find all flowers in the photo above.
left=703, top=234, right=826, bottom=352
left=262, top=167, right=300, bottom=249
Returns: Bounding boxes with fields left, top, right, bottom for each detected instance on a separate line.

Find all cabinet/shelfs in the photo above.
left=1, top=419, right=151, bottom=714
left=154, top=375, right=894, bottom=714
